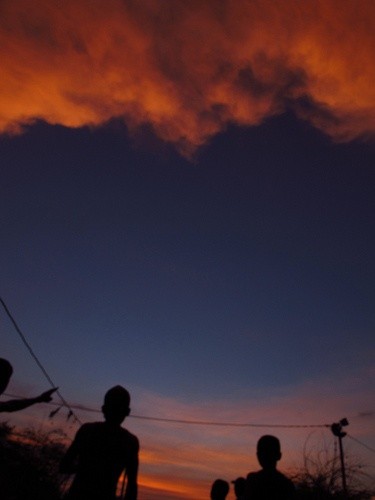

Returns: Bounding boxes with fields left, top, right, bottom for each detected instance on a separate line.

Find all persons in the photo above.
left=0, top=358, right=61, bottom=414
left=45, top=384, right=141, bottom=500
left=232, top=476, right=246, bottom=500
left=209, top=479, right=230, bottom=500
left=245, top=435, right=296, bottom=500
